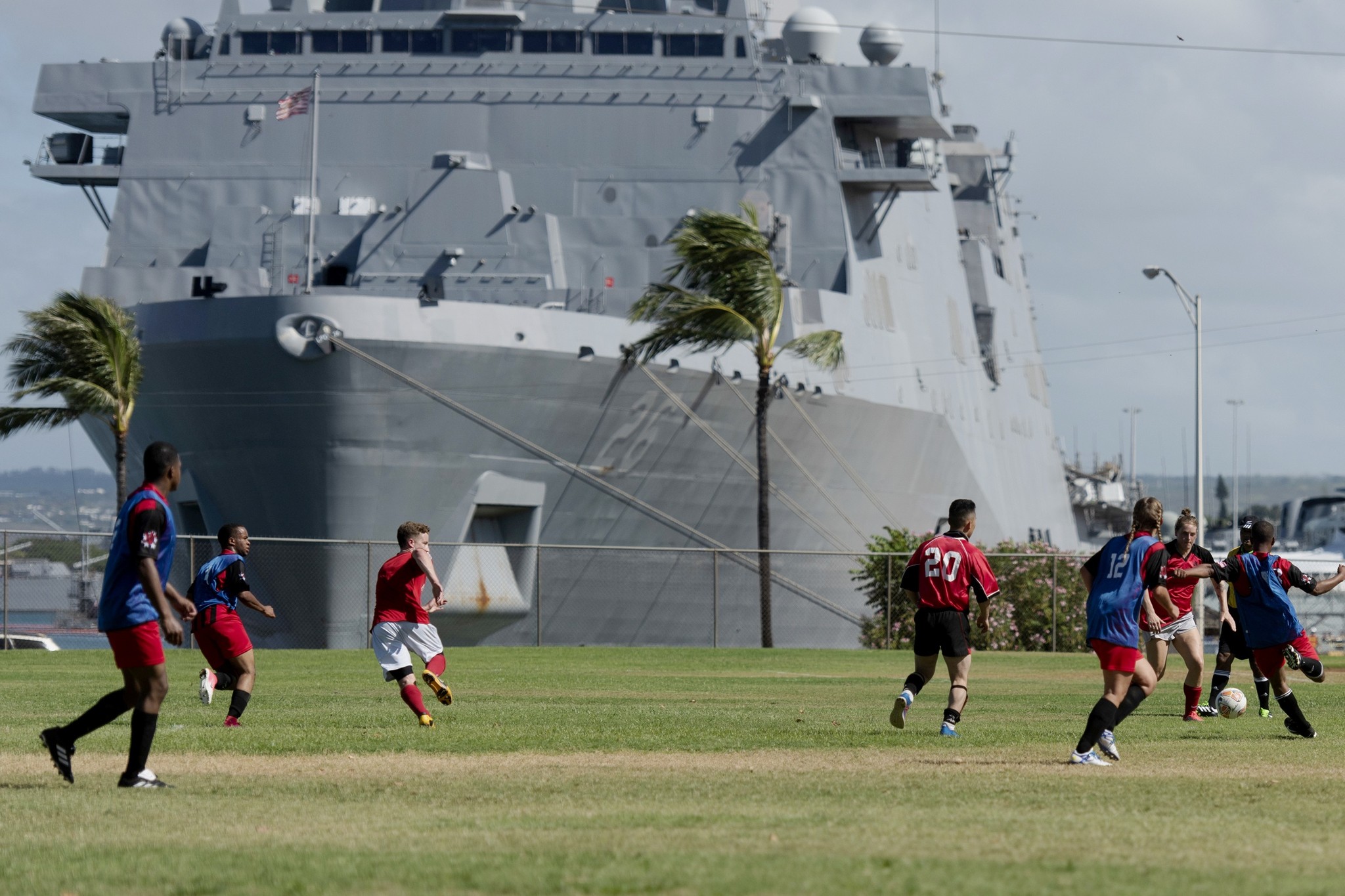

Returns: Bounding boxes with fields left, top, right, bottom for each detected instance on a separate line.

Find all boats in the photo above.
left=1239, top=491, right=1345, bottom=656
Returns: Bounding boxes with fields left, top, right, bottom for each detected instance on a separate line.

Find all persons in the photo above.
left=187, top=522, right=277, bottom=729
left=38, top=440, right=198, bottom=791
left=370, top=521, right=453, bottom=727
left=890, top=499, right=1001, bottom=740
left=1068, top=497, right=1345, bottom=767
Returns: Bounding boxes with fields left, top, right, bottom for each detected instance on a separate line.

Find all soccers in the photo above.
left=1216, top=688, right=1247, bottom=720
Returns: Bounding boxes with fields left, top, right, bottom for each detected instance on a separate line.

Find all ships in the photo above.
left=21, top=0, right=1093, bottom=651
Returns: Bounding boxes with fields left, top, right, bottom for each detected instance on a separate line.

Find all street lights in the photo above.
left=1139, top=264, right=1209, bottom=660
left=1123, top=407, right=1142, bottom=491
left=1226, top=398, right=1249, bottom=549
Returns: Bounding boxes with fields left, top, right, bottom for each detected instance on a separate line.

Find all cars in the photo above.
left=0, top=630, right=60, bottom=651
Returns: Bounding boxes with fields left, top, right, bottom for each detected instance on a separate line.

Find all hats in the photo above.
left=1239, top=515, right=1260, bottom=530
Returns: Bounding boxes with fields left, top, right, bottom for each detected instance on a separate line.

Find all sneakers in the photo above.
left=1097, top=728, right=1121, bottom=761
left=224, top=715, right=242, bottom=727
left=940, top=722, right=957, bottom=736
left=889, top=693, right=913, bottom=729
left=118, top=770, right=176, bottom=789
left=199, top=667, right=218, bottom=707
left=1284, top=717, right=1317, bottom=739
left=422, top=670, right=452, bottom=706
left=1069, top=747, right=1112, bottom=766
left=1197, top=701, right=1218, bottom=718
left=419, top=716, right=434, bottom=728
left=39, top=726, right=76, bottom=784
left=1258, top=707, right=1273, bottom=719
left=1282, top=643, right=1303, bottom=671
left=1183, top=710, right=1202, bottom=722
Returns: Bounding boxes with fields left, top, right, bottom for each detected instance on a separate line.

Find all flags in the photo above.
left=275, top=86, right=312, bottom=121
